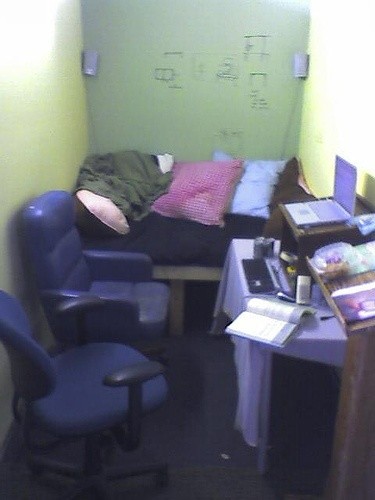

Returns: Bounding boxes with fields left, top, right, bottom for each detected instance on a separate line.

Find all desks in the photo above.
left=231, top=195, right=375, bottom=500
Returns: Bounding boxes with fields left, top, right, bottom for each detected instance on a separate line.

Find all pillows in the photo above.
left=213, top=152, right=287, bottom=221
left=75, top=152, right=172, bottom=237
left=270, top=157, right=320, bottom=214
left=151, top=158, right=246, bottom=227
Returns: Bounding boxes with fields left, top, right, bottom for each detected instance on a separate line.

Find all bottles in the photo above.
left=296, top=275, right=311, bottom=305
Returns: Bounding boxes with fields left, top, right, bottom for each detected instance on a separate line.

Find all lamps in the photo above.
left=84, top=51, right=97, bottom=75
left=293, top=53, right=308, bottom=78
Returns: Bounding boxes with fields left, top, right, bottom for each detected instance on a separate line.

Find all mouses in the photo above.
left=278, top=291, right=293, bottom=302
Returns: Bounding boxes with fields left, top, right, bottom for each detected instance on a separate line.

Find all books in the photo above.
left=225, top=298, right=318, bottom=348
left=330, top=281, right=375, bottom=323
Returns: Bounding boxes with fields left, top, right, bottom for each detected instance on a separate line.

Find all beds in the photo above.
left=72, top=218, right=290, bottom=332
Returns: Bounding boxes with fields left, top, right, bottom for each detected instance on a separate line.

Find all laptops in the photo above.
left=284, top=154, right=358, bottom=228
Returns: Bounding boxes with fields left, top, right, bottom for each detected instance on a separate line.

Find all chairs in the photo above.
left=0, top=291, right=169, bottom=500
left=18, top=189, right=170, bottom=341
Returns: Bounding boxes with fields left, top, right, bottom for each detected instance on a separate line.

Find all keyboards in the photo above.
left=241, top=256, right=274, bottom=294
